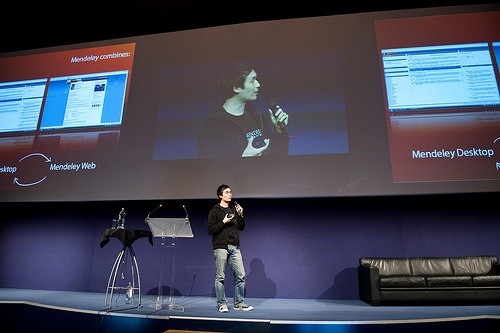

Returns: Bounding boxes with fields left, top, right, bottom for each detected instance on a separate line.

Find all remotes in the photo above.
left=228, top=214, right=233, bottom=218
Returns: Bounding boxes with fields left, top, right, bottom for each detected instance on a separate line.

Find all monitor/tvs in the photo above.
left=0, top=78, right=49, bottom=136
left=39, top=70, right=129, bottom=134
left=379, top=41, right=500, bottom=114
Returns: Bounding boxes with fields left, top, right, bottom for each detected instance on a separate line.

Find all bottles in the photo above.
left=125, top=282, right=134, bottom=308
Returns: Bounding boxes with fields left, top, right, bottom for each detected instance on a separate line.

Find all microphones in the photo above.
left=183, top=205, right=188, bottom=218
left=147, top=205, right=162, bottom=218
left=234, top=201, right=243, bottom=218
left=266, top=97, right=292, bottom=137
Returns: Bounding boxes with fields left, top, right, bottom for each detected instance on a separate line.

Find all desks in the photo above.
left=100, top=229, right=153, bottom=308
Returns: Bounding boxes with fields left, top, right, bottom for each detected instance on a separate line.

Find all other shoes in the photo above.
left=233, top=303, right=253, bottom=311
left=218, top=304, right=229, bottom=312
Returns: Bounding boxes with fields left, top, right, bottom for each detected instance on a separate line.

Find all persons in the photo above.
left=205, top=185, right=254, bottom=313
left=203, top=61, right=290, bottom=197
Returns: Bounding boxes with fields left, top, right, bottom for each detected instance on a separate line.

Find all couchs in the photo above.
left=359, top=256, right=500, bottom=306
